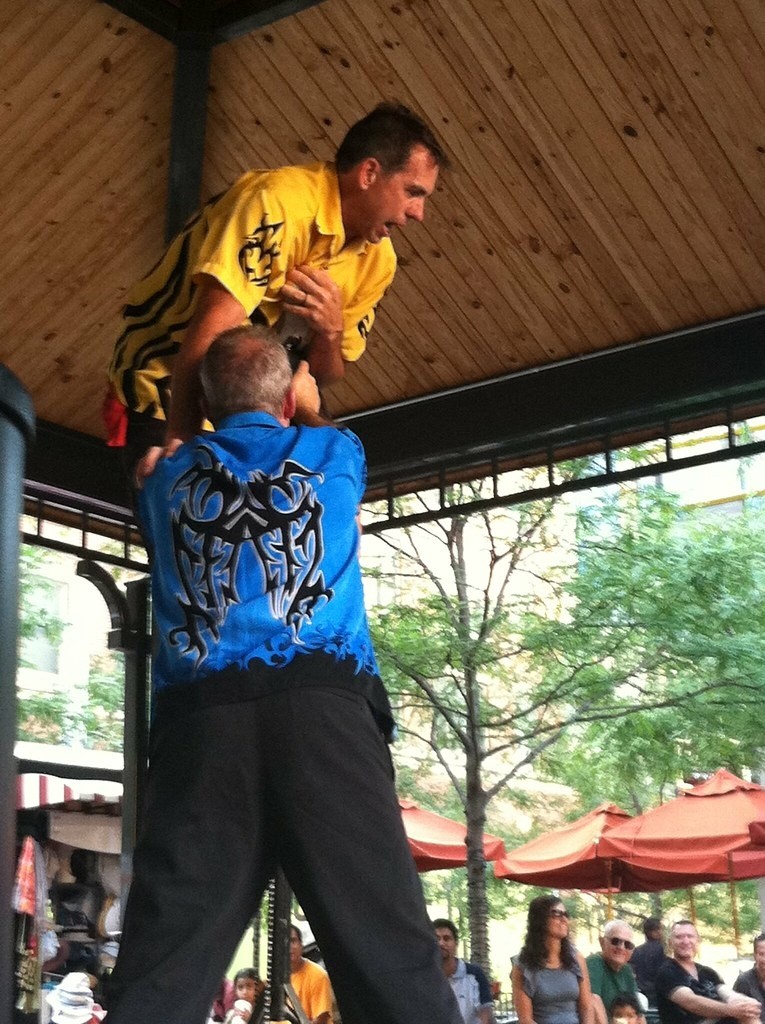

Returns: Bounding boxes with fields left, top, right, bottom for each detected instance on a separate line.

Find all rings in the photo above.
left=300, top=293, right=308, bottom=306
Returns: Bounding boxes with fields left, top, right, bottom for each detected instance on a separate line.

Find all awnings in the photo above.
left=14, top=773, right=125, bottom=814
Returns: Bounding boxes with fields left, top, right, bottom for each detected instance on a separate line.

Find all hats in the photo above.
left=45, top=973, right=94, bottom=1024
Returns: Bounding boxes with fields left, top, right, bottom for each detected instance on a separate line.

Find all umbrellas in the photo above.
left=394, top=768, right=765, bottom=948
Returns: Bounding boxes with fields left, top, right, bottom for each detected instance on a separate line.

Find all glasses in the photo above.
left=546, top=910, right=567, bottom=918
left=605, top=934, right=633, bottom=950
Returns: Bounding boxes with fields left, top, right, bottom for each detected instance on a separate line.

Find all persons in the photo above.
left=104, top=98, right=451, bottom=490
left=510, top=894, right=595, bottom=1024
left=428, top=919, right=494, bottom=1024
left=42, top=849, right=333, bottom=1024
left=585, top=918, right=764, bottom=1023
left=112, top=322, right=460, bottom=1024
